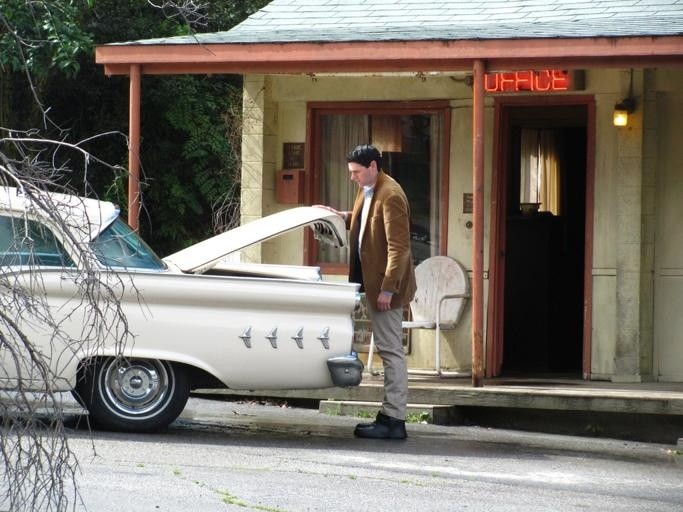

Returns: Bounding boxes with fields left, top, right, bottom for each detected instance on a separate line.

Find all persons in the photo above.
left=310, top=143, right=418, bottom=440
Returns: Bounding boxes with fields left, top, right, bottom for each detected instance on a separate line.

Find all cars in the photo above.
left=0, top=186, right=365, bottom=428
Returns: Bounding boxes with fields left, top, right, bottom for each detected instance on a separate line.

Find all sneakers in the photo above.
left=354, top=410, right=409, bottom=441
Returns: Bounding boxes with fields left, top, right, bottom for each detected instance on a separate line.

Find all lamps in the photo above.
left=611, top=96, right=635, bottom=127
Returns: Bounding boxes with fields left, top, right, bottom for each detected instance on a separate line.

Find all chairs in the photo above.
left=366, top=254, right=472, bottom=380
